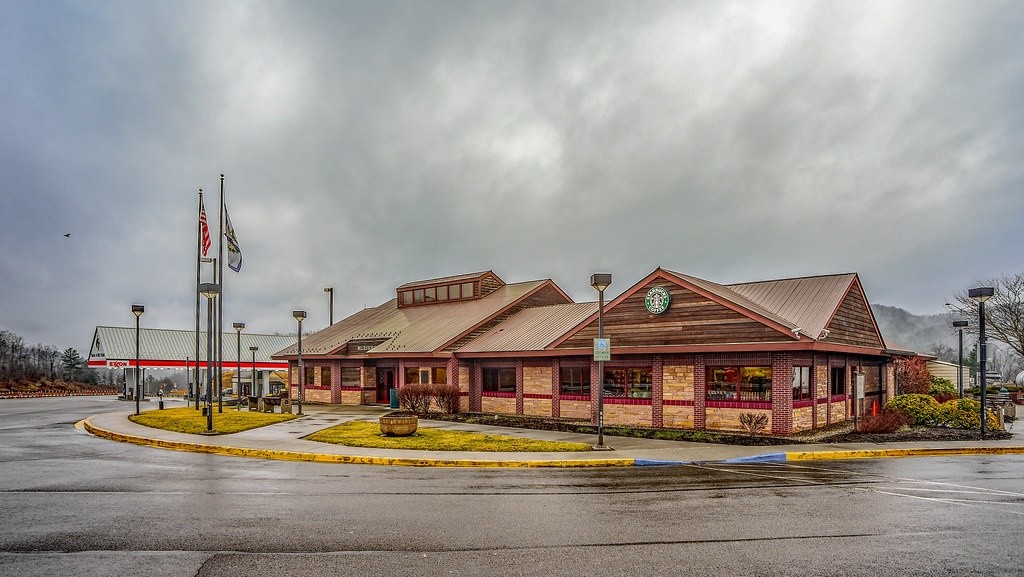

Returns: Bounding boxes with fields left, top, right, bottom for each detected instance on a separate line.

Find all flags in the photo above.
left=225, top=205, right=242, bottom=273
left=200, top=205, right=211, bottom=257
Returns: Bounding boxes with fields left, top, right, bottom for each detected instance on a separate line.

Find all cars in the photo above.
left=222, top=388, right=237, bottom=396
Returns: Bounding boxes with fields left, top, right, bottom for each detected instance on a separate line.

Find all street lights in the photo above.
left=324, top=288, right=333, bottom=326
left=293, top=311, right=307, bottom=416
left=590, top=274, right=612, bottom=446
left=131, top=305, right=145, bottom=415
left=952, top=321, right=969, bottom=400
left=199, top=283, right=221, bottom=430
left=201, top=257, right=217, bottom=397
left=969, top=286, right=995, bottom=440
left=249, top=347, right=258, bottom=396
left=233, top=323, right=245, bottom=404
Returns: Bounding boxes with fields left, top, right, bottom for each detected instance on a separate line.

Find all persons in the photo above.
left=970, top=374, right=974, bottom=386
left=157, top=388, right=164, bottom=400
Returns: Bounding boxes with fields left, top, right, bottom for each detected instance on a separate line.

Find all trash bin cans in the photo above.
left=390, top=389, right=399, bottom=408
